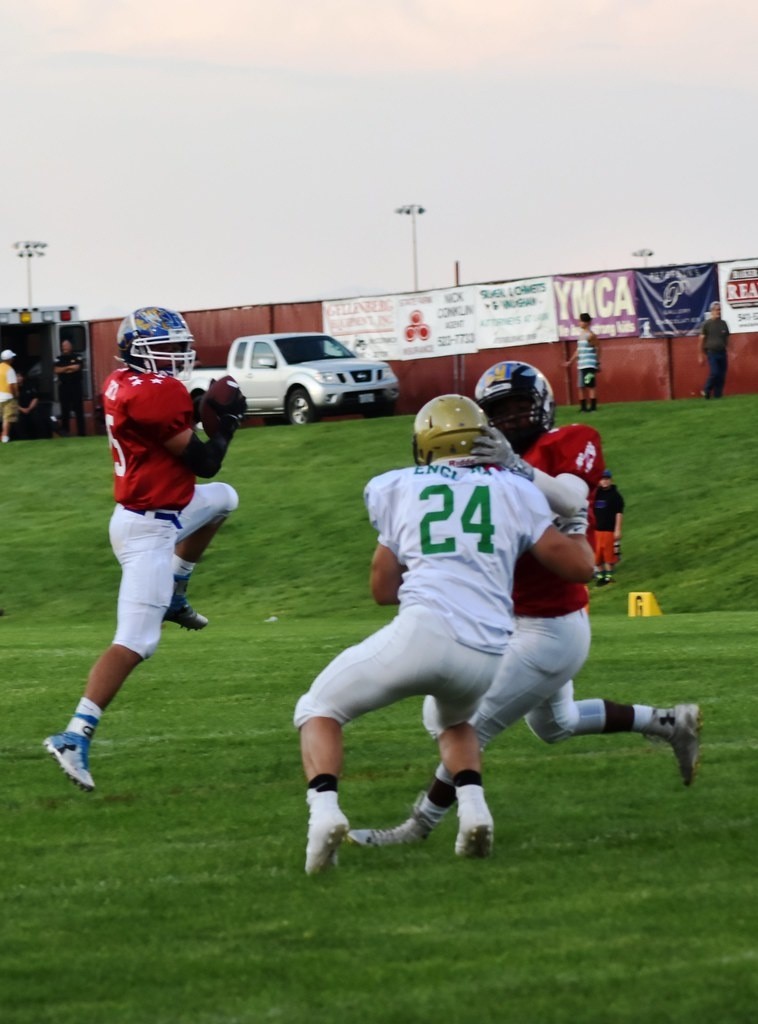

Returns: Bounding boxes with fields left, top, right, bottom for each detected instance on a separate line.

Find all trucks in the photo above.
left=0, top=305, right=94, bottom=437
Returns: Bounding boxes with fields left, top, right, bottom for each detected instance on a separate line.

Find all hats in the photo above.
left=1, top=350, right=16, bottom=361
left=599, top=471, right=612, bottom=478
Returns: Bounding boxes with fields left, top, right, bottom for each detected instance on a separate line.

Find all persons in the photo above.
left=697, top=300, right=731, bottom=401
left=348, top=361, right=702, bottom=849
left=294, top=393, right=595, bottom=874
left=15, top=372, right=46, bottom=437
left=0, top=349, right=24, bottom=444
left=41, top=305, right=246, bottom=793
left=592, top=468, right=625, bottom=587
left=560, top=313, right=602, bottom=413
left=52, top=340, right=87, bottom=438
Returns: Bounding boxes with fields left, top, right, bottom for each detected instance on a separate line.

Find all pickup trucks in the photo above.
left=171, top=331, right=401, bottom=427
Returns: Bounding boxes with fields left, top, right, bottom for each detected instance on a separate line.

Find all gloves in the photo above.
left=471, top=422, right=533, bottom=480
left=209, top=391, right=247, bottom=442
left=556, top=499, right=590, bottom=537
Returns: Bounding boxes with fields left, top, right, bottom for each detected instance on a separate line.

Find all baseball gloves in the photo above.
left=199, top=375, right=248, bottom=439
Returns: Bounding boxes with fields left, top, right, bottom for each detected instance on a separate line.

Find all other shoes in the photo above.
left=594, top=576, right=613, bottom=587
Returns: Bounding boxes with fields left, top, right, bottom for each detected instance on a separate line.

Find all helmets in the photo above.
left=412, top=393, right=490, bottom=467
left=116, top=307, right=190, bottom=379
left=473, top=358, right=555, bottom=448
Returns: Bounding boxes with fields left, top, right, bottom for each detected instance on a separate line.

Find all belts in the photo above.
left=124, top=507, right=183, bottom=529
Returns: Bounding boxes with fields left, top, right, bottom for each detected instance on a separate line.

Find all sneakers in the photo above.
left=160, top=573, right=208, bottom=631
left=668, top=702, right=703, bottom=786
left=346, top=805, right=434, bottom=848
left=304, top=787, right=350, bottom=877
left=454, top=802, right=494, bottom=858
left=42, top=714, right=97, bottom=793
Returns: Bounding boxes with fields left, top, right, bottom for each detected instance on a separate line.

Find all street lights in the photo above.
left=396, top=204, right=426, bottom=291
left=14, top=240, right=47, bottom=306
